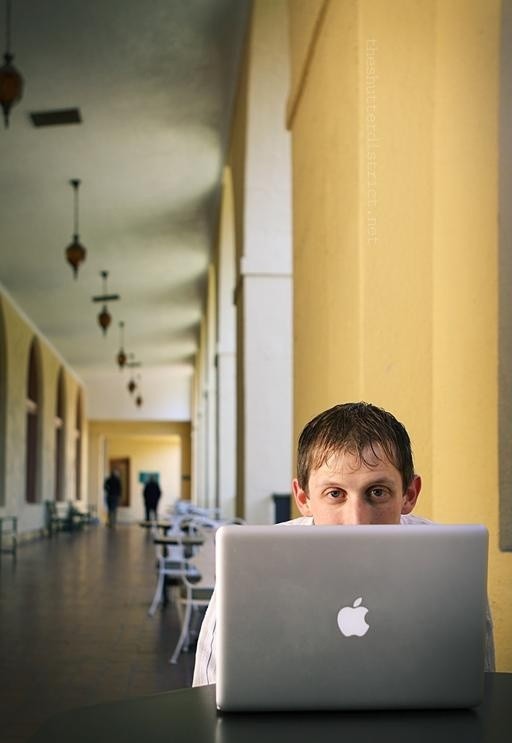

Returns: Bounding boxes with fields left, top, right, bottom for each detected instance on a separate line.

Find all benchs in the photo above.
left=46, top=500, right=90, bottom=537
left=0, top=516, right=18, bottom=553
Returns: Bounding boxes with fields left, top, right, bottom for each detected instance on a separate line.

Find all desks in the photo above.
left=24, top=672, right=512, bottom=743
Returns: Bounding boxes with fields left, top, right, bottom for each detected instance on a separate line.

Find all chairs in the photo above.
left=139, top=501, right=249, bottom=664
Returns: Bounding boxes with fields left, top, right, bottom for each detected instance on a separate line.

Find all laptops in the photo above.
left=215, top=524, right=489, bottom=712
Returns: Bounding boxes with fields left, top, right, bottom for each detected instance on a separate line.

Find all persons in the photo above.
left=103, top=468, right=123, bottom=529
left=190, top=400, right=497, bottom=688
left=142, top=474, right=162, bottom=521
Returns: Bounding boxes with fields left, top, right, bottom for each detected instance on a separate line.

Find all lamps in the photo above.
left=0, top=0, right=23, bottom=128
left=116, top=321, right=128, bottom=374
left=91, top=271, right=120, bottom=336
left=65, top=178, right=87, bottom=281
left=127, top=353, right=137, bottom=394
left=135, top=374, right=143, bottom=407
